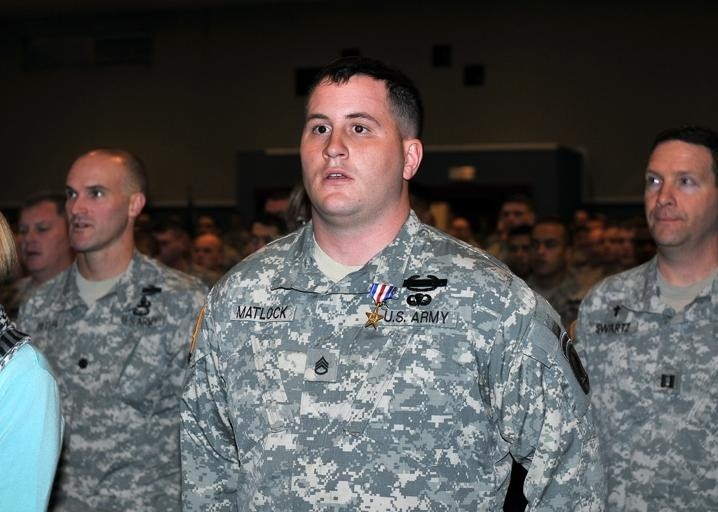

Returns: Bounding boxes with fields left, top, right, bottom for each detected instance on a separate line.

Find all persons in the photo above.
left=567, top=122, right=717, bottom=512
left=131, top=192, right=309, bottom=290
left=422, top=195, right=658, bottom=334
left=1, top=190, right=70, bottom=322
left=1, top=213, right=67, bottom=512
left=12, top=145, right=209, bottom=505
left=173, top=61, right=607, bottom=510
